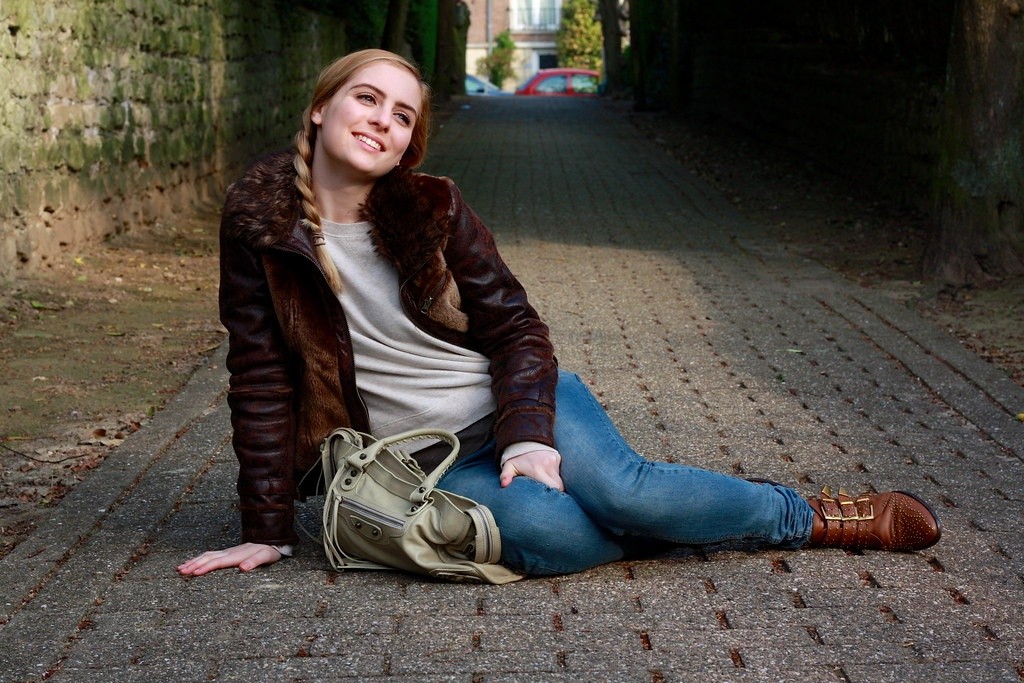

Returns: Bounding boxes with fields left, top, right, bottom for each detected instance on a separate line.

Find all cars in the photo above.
left=464, top=74, right=515, bottom=96
left=515, top=66, right=600, bottom=98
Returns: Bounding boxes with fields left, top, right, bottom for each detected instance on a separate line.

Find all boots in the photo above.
left=805, top=488, right=942, bottom=553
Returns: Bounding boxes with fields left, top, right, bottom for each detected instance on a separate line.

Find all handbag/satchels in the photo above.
left=291, top=426, right=526, bottom=585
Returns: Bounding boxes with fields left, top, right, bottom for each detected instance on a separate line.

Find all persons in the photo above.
left=177, top=48, right=941, bottom=578
